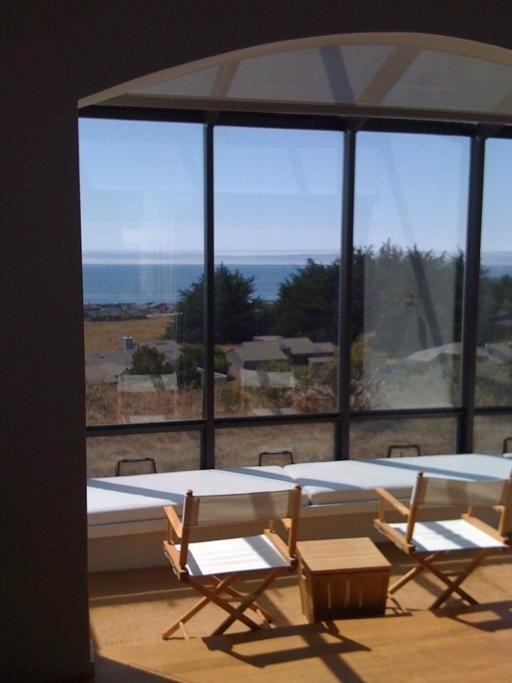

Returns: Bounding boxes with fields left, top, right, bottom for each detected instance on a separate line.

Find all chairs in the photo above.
left=159, top=484, right=303, bottom=644
left=370, top=465, right=511, bottom=616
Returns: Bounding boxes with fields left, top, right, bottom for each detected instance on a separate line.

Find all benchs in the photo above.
left=87, top=451, right=512, bottom=575
